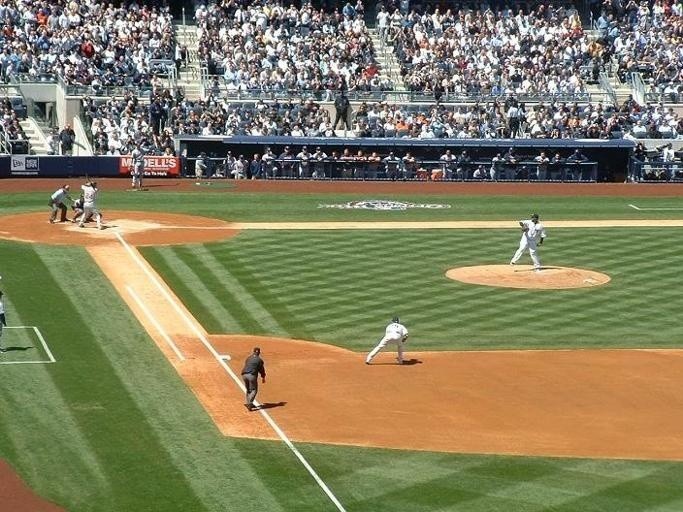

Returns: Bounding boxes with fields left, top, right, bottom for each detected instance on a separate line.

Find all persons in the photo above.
left=71, top=192, right=90, bottom=223
left=240, top=346, right=266, bottom=412
left=48, top=181, right=75, bottom=224
left=509, top=213, right=547, bottom=272
left=0, top=0, right=682, bottom=181
left=365, top=316, right=410, bottom=364
left=78, top=178, right=106, bottom=231
left=129, top=141, right=149, bottom=190
left=0, top=290, right=8, bottom=351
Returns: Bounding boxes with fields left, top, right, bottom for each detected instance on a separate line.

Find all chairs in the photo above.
left=0, top=0, right=682, bottom=188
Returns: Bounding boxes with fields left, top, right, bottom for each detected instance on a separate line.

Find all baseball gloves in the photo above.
left=522, top=224, right=528, bottom=231
